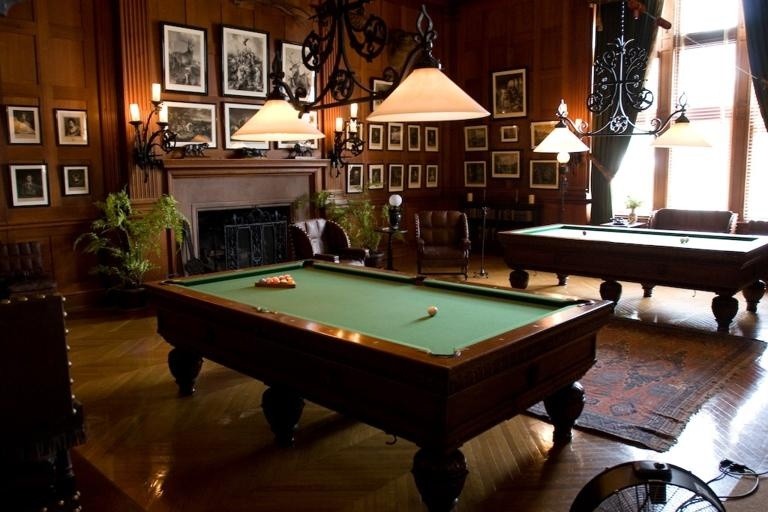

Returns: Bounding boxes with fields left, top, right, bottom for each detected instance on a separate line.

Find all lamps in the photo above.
left=230, top=1, right=491, bottom=141
left=328, top=103, right=366, bottom=178
left=388, top=193, right=403, bottom=230
left=129, top=83, right=176, bottom=184
left=532, top=1, right=712, bottom=163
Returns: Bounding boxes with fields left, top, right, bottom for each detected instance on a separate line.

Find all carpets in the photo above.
left=522, top=314, right=768, bottom=453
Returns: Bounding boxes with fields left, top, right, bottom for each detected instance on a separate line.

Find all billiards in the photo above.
left=260, top=274, right=295, bottom=285
left=428, top=306, right=437, bottom=316
left=680, top=238, right=688, bottom=243
left=583, top=231, right=586, bottom=235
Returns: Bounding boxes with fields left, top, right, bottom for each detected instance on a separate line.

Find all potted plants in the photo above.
left=626, top=194, right=641, bottom=224
left=72, top=183, right=191, bottom=309
left=346, top=173, right=386, bottom=271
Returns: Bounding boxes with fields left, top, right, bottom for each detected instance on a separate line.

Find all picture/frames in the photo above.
left=346, top=80, right=439, bottom=195
left=462, top=68, right=566, bottom=190
left=160, top=23, right=319, bottom=150
left=5, top=105, right=91, bottom=208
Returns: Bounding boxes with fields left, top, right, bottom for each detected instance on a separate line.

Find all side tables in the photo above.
left=373, top=227, right=408, bottom=271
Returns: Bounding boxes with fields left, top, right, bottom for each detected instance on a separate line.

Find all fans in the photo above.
left=568, top=460, right=726, bottom=511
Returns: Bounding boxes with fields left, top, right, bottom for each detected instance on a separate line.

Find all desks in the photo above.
left=142, top=259, right=613, bottom=511
left=495, top=222, right=768, bottom=332
left=600, top=218, right=647, bottom=228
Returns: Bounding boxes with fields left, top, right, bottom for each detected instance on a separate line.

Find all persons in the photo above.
left=24, top=175, right=39, bottom=195
left=65, top=118, right=81, bottom=136
left=18, top=113, right=32, bottom=129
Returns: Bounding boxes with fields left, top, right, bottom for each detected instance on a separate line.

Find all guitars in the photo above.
left=182, top=220, right=205, bottom=275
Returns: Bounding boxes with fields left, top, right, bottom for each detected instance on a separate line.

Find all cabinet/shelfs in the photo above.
left=459, top=201, right=543, bottom=250
left=0, top=291, right=86, bottom=512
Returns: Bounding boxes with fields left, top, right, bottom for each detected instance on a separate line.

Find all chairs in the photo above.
left=288, top=218, right=366, bottom=268
left=646, top=208, right=739, bottom=234
left=414, top=210, right=471, bottom=280
left=0, top=241, right=57, bottom=301
